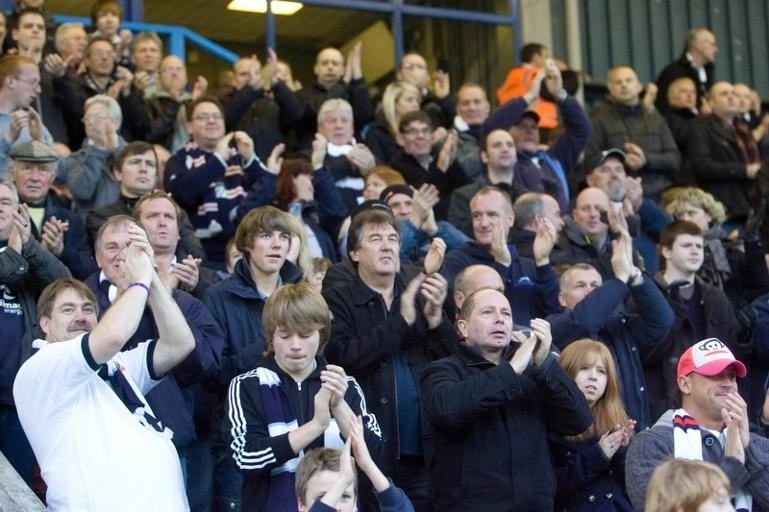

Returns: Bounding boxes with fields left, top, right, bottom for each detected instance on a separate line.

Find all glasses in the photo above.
left=402, top=126, right=432, bottom=133
left=15, top=76, right=39, bottom=89
left=92, top=50, right=117, bottom=59
left=81, top=116, right=108, bottom=124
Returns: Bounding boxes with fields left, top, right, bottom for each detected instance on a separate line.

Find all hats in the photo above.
left=380, top=184, right=414, bottom=204
left=585, top=149, right=625, bottom=174
left=677, top=338, right=746, bottom=378
left=512, top=110, right=540, bottom=125
left=9, top=139, right=59, bottom=161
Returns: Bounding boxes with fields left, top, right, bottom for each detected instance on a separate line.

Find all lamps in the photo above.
left=226, top=0, right=303, bottom=17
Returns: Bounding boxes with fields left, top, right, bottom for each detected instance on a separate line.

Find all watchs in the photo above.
left=628, top=263, right=643, bottom=284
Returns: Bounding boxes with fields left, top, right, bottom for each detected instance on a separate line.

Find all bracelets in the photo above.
left=128, top=281, right=152, bottom=298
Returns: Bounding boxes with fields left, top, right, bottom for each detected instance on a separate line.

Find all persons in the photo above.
left=168, top=95, right=277, bottom=214
left=295, top=415, right=417, bottom=512
left=201, top=206, right=324, bottom=512
left=375, top=79, right=421, bottom=134
left=89, top=0, right=129, bottom=60
left=70, top=34, right=152, bottom=150
left=477, top=62, right=591, bottom=194
left=81, top=214, right=227, bottom=512
left=388, top=109, right=474, bottom=222
left=508, top=191, right=570, bottom=269
left=443, top=127, right=539, bottom=234
left=625, top=337, right=769, bottom=512
left=309, top=257, right=333, bottom=291
left=135, top=189, right=220, bottom=299
left=379, top=183, right=473, bottom=265
left=87, top=140, right=204, bottom=270
left=733, top=79, right=766, bottom=178
left=224, top=234, right=245, bottom=276
left=495, top=43, right=580, bottom=157
left=295, top=39, right=378, bottom=144
left=675, top=185, right=743, bottom=303
left=562, top=185, right=637, bottom=279
left=643, top=455, right=737, bottom=512
left=128, top=32, right=162, bottom=89
left=0, top=176, right=74, bottom=485
left=418, top=287, right=593, bottom=512
left=62, top=93, right=133, bottom=214
left=50, top=18, right=92, bottom=80
left=694, top=79, right=762, bottom=228
left=234, top=140, right=337, bottom=265
left=318, top=211, right=448, bottom=511
left=449, top=264, right=556, bottom=358
left=11, top=6, right=63, bottom=143
left=583, top=65, right=679, bottom=201
left=143, top=55, right=208, bottom=148
left=397, top=54, right=461, bottom=127
left=452, top=78, right=493, bottom=127
left=0, top=54, right=55, bottom=177
left=662, top=77, right=710, bottom=186
left=227, top=282, right=398, bottom=512
left=262, top=56, right=301, bottom=108
left=543, top=228, right=680, bottom=435
left=334, top=165, right=408, bottom=263
left=278, top=210, right=318, bottom=289
left=553, top=337, right=639, bottom=512
left=14, top=223, right=195, bottom=512
left=651, top=26, right=717, bottom=119
left=441, top=185, right=567, bottom=323
left=300, top=92, right=385, bottom=189
left=648, top=219, right=760, bottom=384
left=584, top=147, right=672, bottom=274
left=1, top=10, right=12, bottom=73
left=224, top=47, right=304, bottom=164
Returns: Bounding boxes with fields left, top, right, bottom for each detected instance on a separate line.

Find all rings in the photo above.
left=22, top=222, right=28, bottom=228
left=144, top=240, right=151, bottom=246
